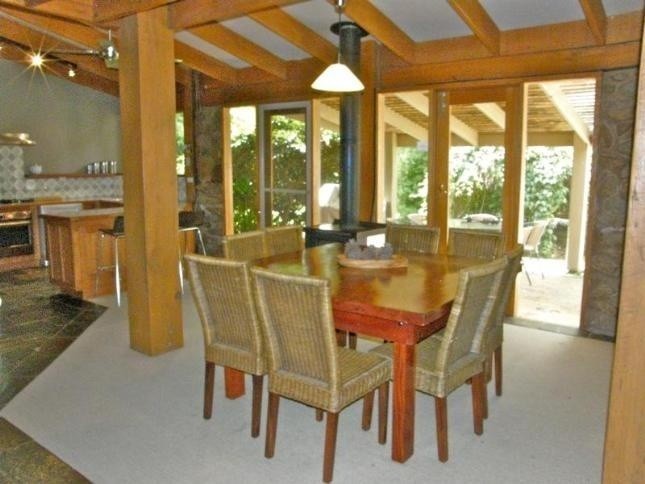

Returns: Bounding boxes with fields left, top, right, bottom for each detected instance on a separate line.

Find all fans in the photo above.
left=33, top=29, right=184, bottom=70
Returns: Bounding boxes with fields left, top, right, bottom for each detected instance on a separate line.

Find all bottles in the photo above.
left=102, top=161, right=108, bottom=174
left=87, top=165, right=92, bottom=174
left=93, top=162, right=100, bottom=174
left=110, top=160, right=117, bottom=173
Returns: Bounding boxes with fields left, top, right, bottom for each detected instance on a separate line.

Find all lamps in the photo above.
left=309, top=0, right=366, bottom=94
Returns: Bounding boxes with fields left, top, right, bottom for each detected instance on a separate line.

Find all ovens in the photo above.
left=0, top=210, right=33, bottom=258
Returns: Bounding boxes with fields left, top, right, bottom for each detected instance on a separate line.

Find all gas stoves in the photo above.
left=0, top=198, right=36, bottom=205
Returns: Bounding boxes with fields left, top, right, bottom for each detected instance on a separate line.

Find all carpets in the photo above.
left=0, top=277, right=616, bottom=484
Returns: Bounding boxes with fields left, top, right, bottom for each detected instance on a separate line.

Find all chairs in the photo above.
left=465, top=245, right=523, bottom=397
left=182, top=254, right=322, bottom=437
left=179, top=209, right=208, bottom=294
left=247, top=265, right=392, bottom=483
left=363, top=256, right=508, bottom=462
left=94, top=215, right=128, bottom=307
left=449, top=228, right=504, bottom=259
left=520, top=221, right=548, bottom=286
left=221, top=230, right=268, bottom=260
left=266, top=225, right=304, bottom=254
left=386, top=224, right=439, bottom=254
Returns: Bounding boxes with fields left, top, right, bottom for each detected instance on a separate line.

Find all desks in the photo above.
left=226, top=242, right=493, bottom=461
